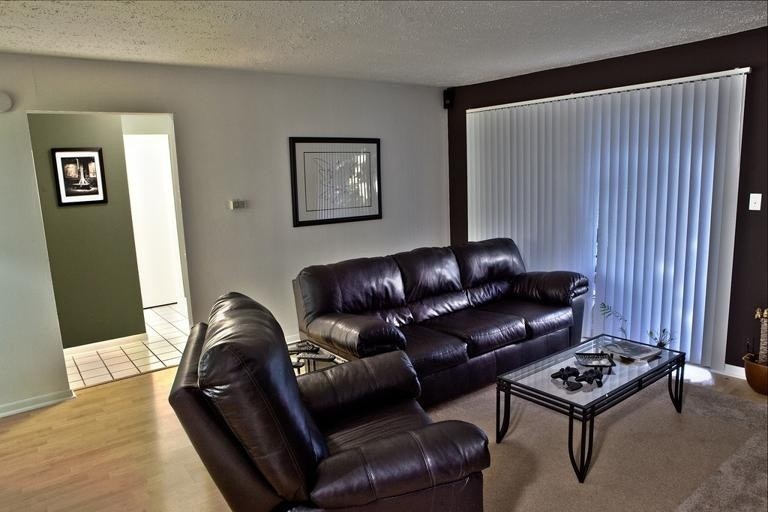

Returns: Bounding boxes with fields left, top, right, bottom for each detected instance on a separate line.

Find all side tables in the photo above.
left=287, top=339, right=355, bottom=380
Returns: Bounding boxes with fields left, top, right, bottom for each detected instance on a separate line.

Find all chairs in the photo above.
left=168, top=291, right=491, bottom=512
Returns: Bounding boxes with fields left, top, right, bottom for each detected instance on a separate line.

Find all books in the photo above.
left=599, top=339, right=663, bottom=362
left=633, top=355, right=661, bottom=364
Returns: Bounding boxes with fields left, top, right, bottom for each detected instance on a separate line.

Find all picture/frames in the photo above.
left=51, top=147, right=108, bottom=207
left=289, top=136, right=382, bottom=227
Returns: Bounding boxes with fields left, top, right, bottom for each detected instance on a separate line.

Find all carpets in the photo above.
left=423, top=379, right=767, bottom=512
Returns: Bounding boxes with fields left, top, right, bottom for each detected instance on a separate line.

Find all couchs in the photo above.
left=292, top=238, right=589, bottom=410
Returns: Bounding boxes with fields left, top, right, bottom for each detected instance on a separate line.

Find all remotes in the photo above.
left=297, top=352, right=336, bottom=362
left=288, top=345, right=319, bottom=353
